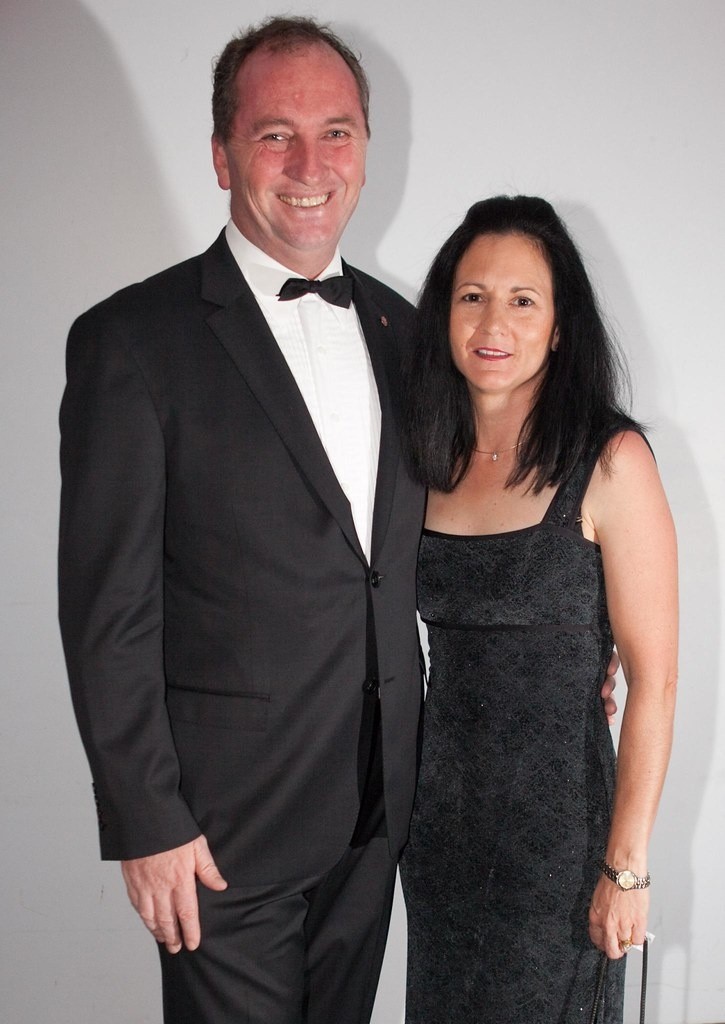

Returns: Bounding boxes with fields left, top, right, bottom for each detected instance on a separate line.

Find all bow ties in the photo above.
left=276, top=271, right=355, bottom=309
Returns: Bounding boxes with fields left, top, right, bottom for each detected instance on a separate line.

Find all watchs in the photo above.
left=599, top=857, right=650, bottom=892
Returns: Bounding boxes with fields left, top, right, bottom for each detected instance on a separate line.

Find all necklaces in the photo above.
left=473, top=440, right=525, bottom=462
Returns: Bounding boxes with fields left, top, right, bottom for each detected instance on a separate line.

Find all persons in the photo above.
left=57, top=18, right=620, bottom=1024
left=399, top=195, right=678, bottom=1024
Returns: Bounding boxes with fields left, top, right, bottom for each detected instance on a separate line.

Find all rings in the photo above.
left=618, top=936, right=633, bottom=953
